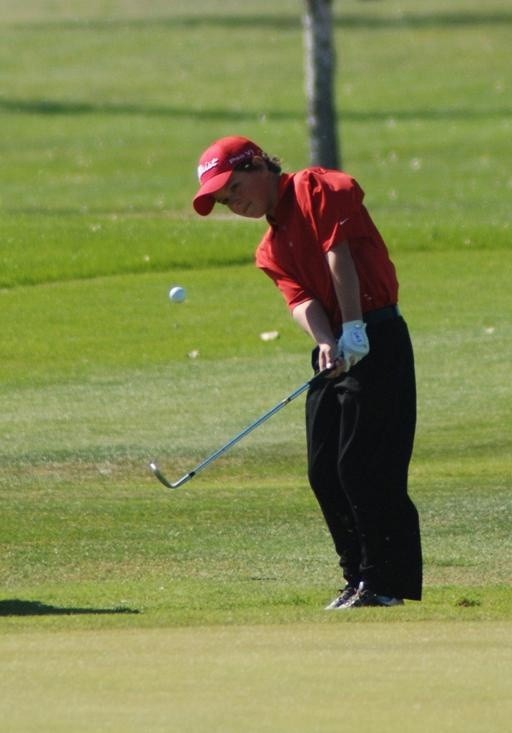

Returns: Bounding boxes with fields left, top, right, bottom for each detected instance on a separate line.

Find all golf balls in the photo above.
left=169, top=287, right=186, bottom=302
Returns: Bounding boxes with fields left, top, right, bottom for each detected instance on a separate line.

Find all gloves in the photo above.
left=335, top=320, right=369, bottom=374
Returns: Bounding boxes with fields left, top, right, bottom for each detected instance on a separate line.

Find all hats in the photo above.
left=192, top=135, right=263, bottom=217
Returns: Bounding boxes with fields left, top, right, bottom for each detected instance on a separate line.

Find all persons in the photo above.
left=193, top=136, right=423, bottom=609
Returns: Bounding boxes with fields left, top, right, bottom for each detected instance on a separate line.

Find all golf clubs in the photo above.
left=149, top=353, right=344, bottom=489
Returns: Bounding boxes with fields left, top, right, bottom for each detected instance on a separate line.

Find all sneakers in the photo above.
left=324, top=581, right=405, bottom=610
